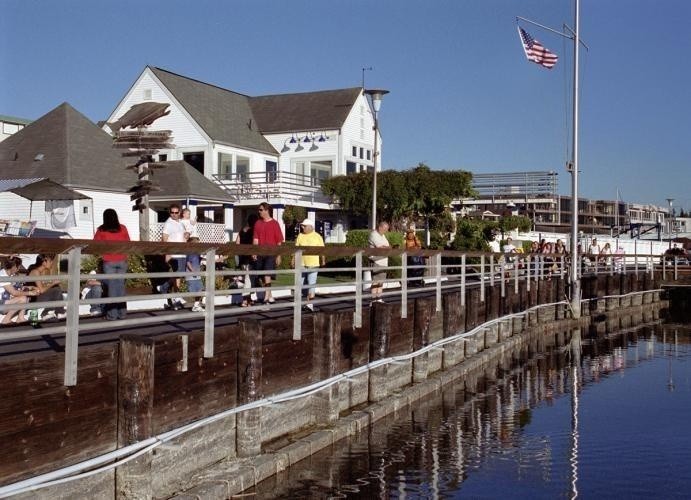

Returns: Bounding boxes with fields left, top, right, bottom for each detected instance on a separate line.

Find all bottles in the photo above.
left=29, top=308, right=39, bottom=327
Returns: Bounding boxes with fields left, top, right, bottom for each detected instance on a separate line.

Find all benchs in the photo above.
left=0, top=275, right=503, bottom=327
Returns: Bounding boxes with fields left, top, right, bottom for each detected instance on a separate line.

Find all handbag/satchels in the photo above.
left=2, top=283, right=21, bottom=300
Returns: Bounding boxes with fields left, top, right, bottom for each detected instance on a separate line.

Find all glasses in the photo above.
left=259, top=208, right=263, bottom=211
left=170, top=211, right=179, bottom=214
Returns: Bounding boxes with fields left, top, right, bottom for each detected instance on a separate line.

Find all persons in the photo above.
left=366, top=220, right=394, bottom=304
left=290, top=217, right=325, bottom=313
left=91, top=205, right=130, bottom=319
left=183, top=238, right=207, bottom=313
left=0, top=257, right=41, bottom=324
left=176, top=208, right=198, bottom=242
left=154, top=205, right=191, bottom=306
left=403, top=226, right=421, bottom=281
left=503, top=236, right=625, bottom=277
left=235, top=212, right=254, bottom=307
left=26, top=253, right=68, bottom=321
left=251, top=202, right=283, bottom=306
left=79, top=259, right=108, bottom=317
left=670, top=243, right=681, bottom=264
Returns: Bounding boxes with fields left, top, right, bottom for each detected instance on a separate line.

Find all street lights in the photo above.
left=666, top=197, right=676, bottom=252
left=364, top=87, right=390, bottom=232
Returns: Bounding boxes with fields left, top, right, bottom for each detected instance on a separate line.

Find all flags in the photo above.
left=518, top=25, right=559, bottom=70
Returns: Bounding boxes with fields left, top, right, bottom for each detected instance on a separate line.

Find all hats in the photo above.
left=300, top=219, right=313, bottom=225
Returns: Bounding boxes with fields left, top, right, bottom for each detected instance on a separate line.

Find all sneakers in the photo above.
left=192, top=304, right=205, bottom=312
left=304, top=305, right=314, bottom=312
left=157, top=285, right=167, bottom=294
